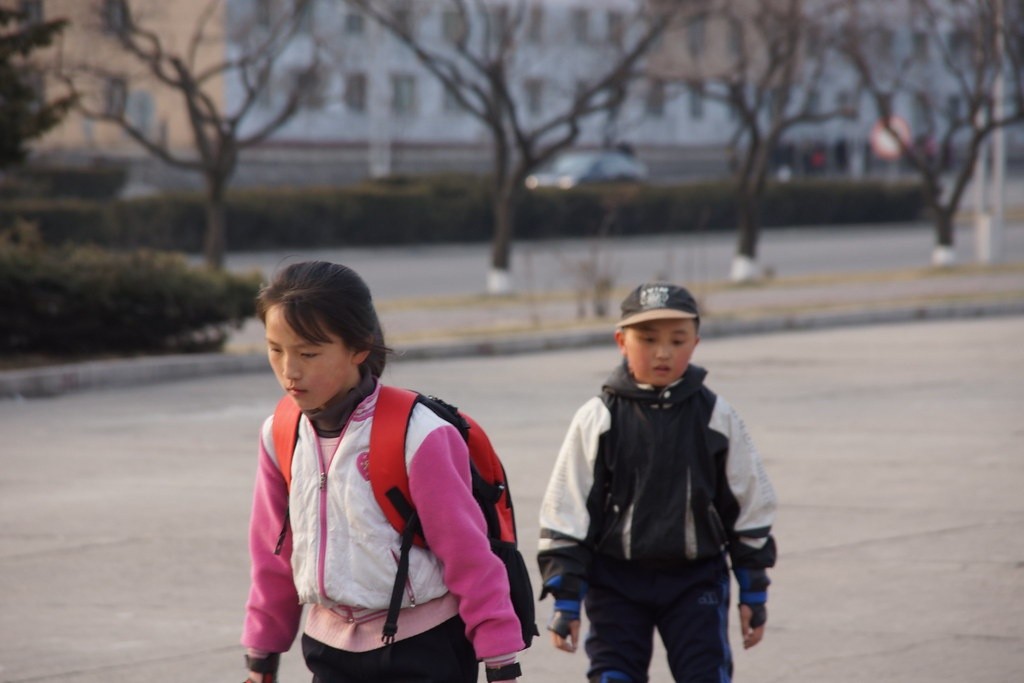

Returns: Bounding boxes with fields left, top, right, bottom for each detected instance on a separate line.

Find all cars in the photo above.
left=749, top=136, right=872, bottom=183
left=522, top=147, right=651, bottom=192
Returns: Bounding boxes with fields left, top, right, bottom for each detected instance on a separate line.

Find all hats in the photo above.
left=618, top=283, right=701, bottom=330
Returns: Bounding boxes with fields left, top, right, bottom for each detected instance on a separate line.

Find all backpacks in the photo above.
left=274, top=385, right=539, bottom=651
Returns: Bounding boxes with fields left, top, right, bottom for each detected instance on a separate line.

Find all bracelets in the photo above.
left=485, top=660, right=523, bottom=683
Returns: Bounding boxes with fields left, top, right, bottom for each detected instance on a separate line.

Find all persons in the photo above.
left=240, top=262, right=524, bottom=683
left=536, top=283, right=778, bottom=683
